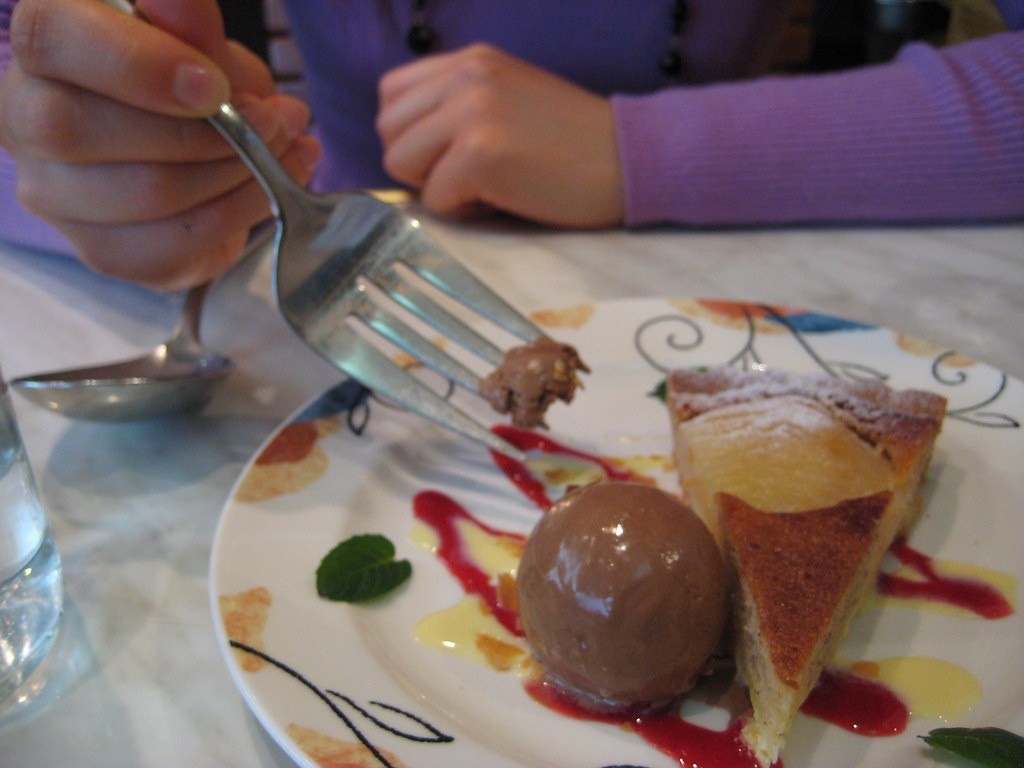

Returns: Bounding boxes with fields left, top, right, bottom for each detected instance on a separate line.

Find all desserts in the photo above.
left=514, top=364, right=949, bottom=768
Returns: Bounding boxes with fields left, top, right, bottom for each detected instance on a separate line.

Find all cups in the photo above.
left=0, top=536, right=65, bottom=704
left=0, top=372, right=63, bottom=578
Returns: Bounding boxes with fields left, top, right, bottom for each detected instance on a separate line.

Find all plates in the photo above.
left=209, top=302, right=1024, bottom=768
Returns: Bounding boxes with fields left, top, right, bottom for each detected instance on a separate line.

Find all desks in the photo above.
left=0, top=191, right=1024, bottom=768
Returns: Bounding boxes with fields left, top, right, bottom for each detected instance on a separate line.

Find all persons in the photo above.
left=0, top=0, right=1024, bottom=294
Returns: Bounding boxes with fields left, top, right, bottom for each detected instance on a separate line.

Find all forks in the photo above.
left=101, top=0, right=592, bottom=457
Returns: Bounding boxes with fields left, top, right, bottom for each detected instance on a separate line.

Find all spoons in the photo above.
left=8, top=216, right=277, bottom=421
